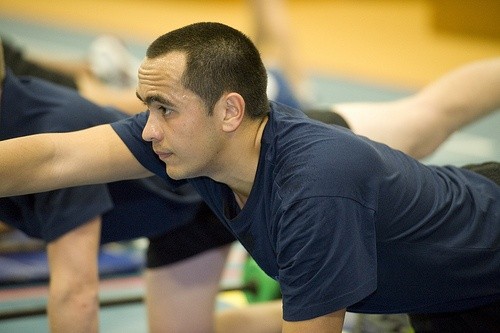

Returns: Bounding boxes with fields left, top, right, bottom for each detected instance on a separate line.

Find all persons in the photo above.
left=1, top=21, right=499, bottom=333
left=0, top=34, right=500, bottom=333
